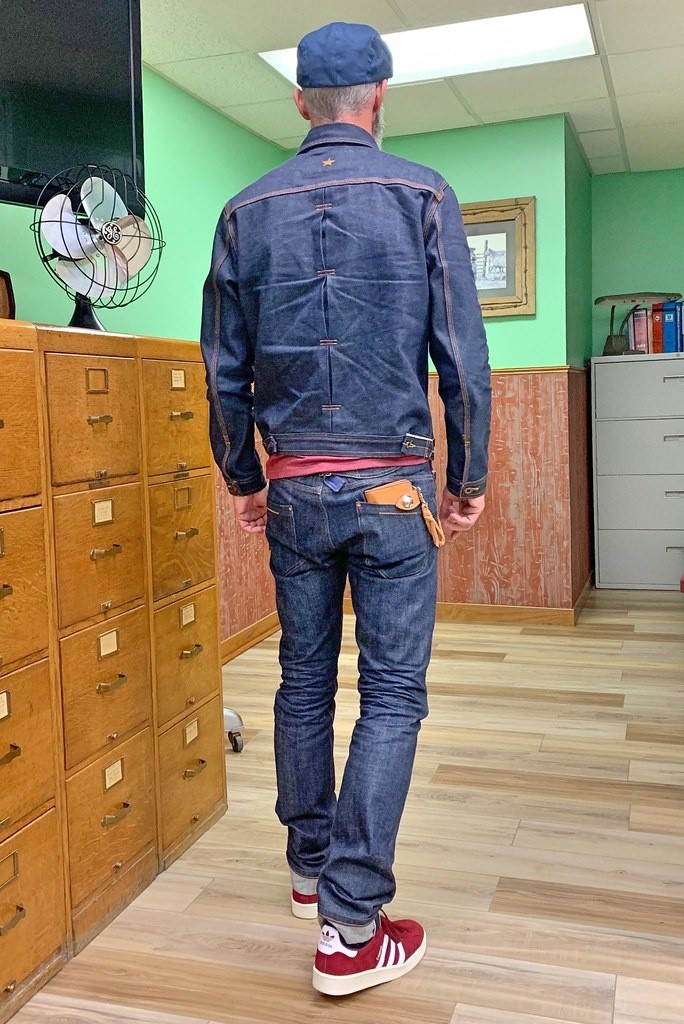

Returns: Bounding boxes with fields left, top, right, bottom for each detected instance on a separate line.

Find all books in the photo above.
left=629, top=301, right=684, bottom=355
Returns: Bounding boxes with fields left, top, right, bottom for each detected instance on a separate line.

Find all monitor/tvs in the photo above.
left=0, top=0, right=146, bottom=223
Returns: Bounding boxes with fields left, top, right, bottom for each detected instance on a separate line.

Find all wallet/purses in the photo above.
left=364, top=479, right=446, bottom=548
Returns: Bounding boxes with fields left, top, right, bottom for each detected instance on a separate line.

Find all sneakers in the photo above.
left=312, top=909, right=426, bottom=995
left=291, top=889, right=319, bottom=919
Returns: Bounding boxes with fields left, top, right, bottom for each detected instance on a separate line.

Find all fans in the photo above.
left=31, top=163, right=166, bottom=332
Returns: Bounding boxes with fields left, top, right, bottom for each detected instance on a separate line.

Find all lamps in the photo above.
left=595, top=292, right=682, bottom=356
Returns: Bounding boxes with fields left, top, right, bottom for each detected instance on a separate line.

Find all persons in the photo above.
left=201, top=22, right=493, bottom=997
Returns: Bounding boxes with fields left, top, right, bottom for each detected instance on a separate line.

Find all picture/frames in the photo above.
left=459, top=196, right=535, bottom=316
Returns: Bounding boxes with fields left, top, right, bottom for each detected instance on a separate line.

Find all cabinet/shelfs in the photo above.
left=0, top=320, right=228, bottom=1024
left=591, top=352, right=684, bottom=591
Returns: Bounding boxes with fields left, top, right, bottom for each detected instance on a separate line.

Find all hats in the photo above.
left=297, top=22, right=393, bottom=88
